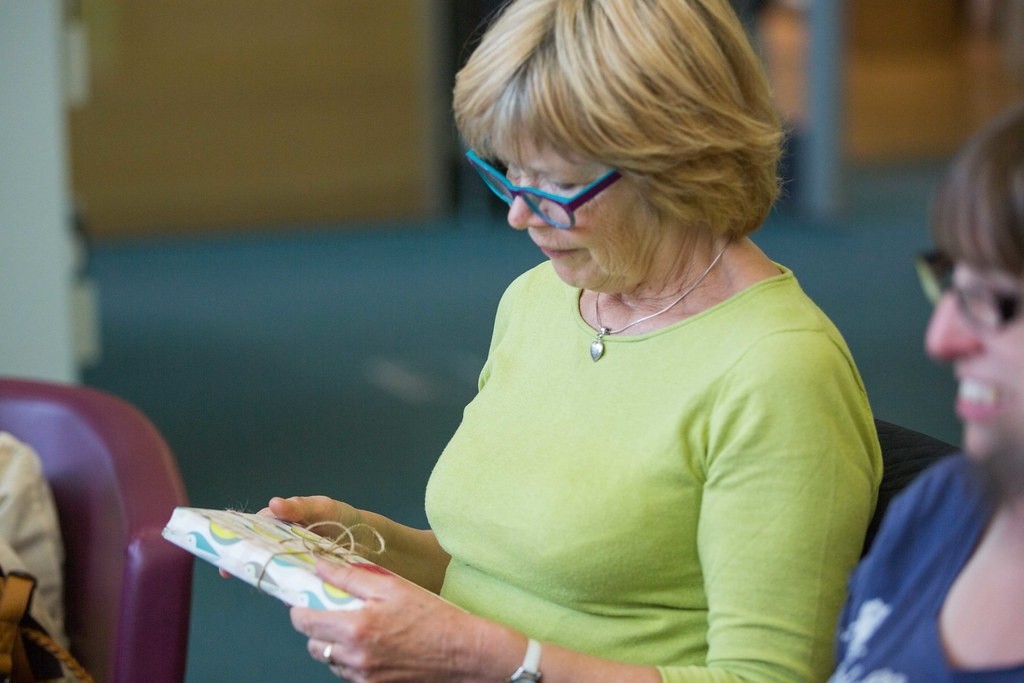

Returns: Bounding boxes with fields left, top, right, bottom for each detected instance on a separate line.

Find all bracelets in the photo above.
left=511, top=639, right=542, bottom=683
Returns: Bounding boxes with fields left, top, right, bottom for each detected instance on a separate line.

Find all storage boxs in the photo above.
left=162, top=507, right=472, bottom=613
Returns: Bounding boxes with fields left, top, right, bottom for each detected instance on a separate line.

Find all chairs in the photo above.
left=0, top=379, right=194, bottom=683
left=859, top=418, right=965, bottom=560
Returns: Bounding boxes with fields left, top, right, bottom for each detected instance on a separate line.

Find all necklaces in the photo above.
left=590, top=233, right=736, bottom=360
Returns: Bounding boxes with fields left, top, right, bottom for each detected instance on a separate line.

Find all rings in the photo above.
left=324, top=642, right=333, bottom=665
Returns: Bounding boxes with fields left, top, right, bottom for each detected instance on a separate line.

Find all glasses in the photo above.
left=914, top=250, right=1021, bottom=334
left=465, top=150, right=622, bottom=229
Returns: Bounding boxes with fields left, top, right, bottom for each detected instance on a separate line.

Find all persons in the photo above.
left=0, top=430, right=75, bottom=683
left=830, top=111, right=1024, bottom=683
left=217, top=0, right=884, bottom=683
